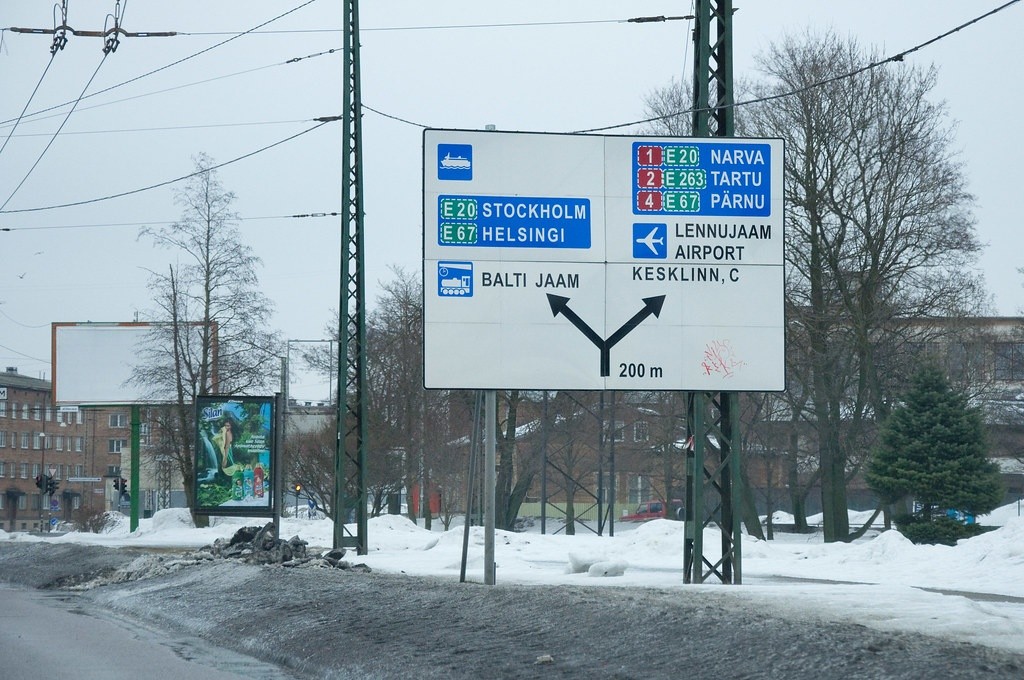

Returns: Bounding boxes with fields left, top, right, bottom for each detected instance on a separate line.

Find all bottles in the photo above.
left=254, top=464, right=264, bottom=498
left=232, top=465, right=243, bottom=501
left=244, top=464, right=254, bottom=498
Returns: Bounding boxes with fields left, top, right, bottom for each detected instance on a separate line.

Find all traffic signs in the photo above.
left=424, top=128, right=787, bottom=391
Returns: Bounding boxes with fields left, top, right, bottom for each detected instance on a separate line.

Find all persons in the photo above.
left=223, top=418, right=242, bottom=466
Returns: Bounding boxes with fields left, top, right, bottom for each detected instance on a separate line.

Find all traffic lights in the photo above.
left=296, top=484, right=300, bottom=495
left=119, top=477, right=128, bottom=496
left=43, top=475, right=53, bottom=494
left=49, top=480, right=60, bottom=496
left=36, top=474, right=46, bottom=495
left=113, top=477, right=120, bottom=491
